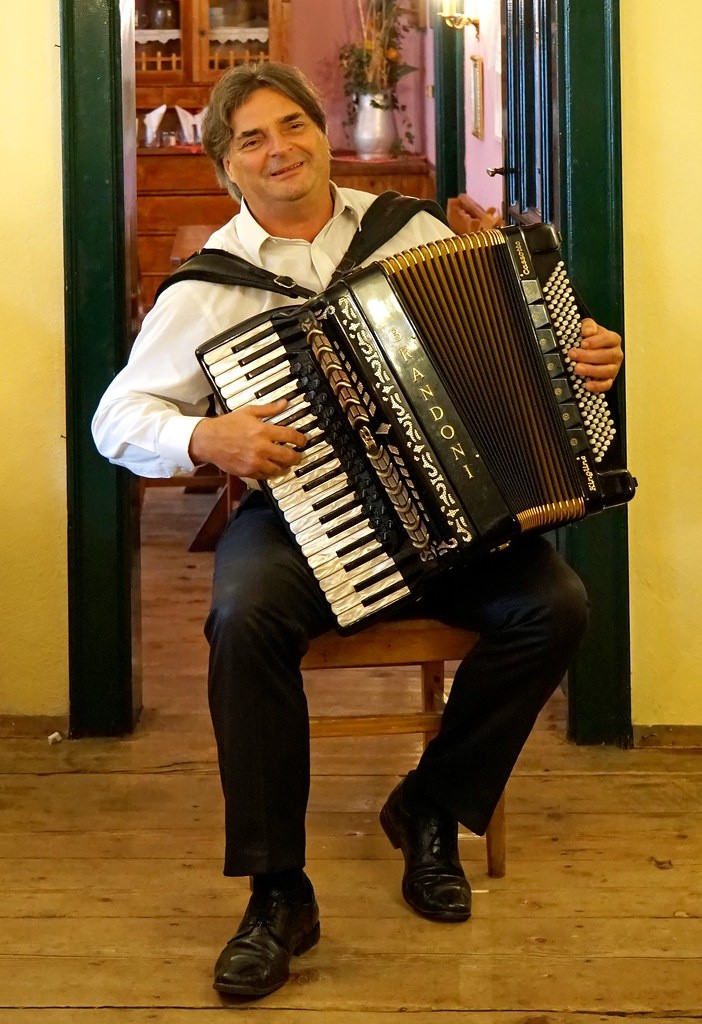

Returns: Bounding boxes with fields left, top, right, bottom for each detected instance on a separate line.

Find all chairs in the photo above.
left=224, top=470, right=515, bottom=877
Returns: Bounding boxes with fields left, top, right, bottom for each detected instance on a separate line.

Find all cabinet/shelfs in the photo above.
left=135, top=0, right=292, bottom=112
left=131, top=141, right=436, bottom=333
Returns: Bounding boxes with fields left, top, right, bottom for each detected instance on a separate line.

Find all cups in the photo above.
left=145, top=132, right=160, bottom=148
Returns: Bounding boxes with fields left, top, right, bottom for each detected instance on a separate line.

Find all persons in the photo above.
left=89, top=61, right=625, bottom=1003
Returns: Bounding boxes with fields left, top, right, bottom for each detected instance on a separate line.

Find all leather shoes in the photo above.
left=210, top=867, right=322, bottom=998
left=377, top=767, right=472, bottom=923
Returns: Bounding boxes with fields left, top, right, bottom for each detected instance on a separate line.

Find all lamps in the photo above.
left=437, top=0, right=481, bottom=40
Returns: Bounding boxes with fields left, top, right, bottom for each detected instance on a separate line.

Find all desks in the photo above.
left=169, top=222, right=252, bottom=557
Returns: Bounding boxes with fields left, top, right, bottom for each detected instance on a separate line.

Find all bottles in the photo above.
left=162, top=132, right=169, bottom=146
left=169, top=132, right=176, bottom=146
left=209, top=8, right=224, bottom=27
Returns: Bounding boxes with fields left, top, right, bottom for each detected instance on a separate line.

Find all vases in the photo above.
left=352, top=88, right=400, bottom=161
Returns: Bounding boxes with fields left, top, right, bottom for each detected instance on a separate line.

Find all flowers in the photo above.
left=340, top=1, right=416, bottom=95
